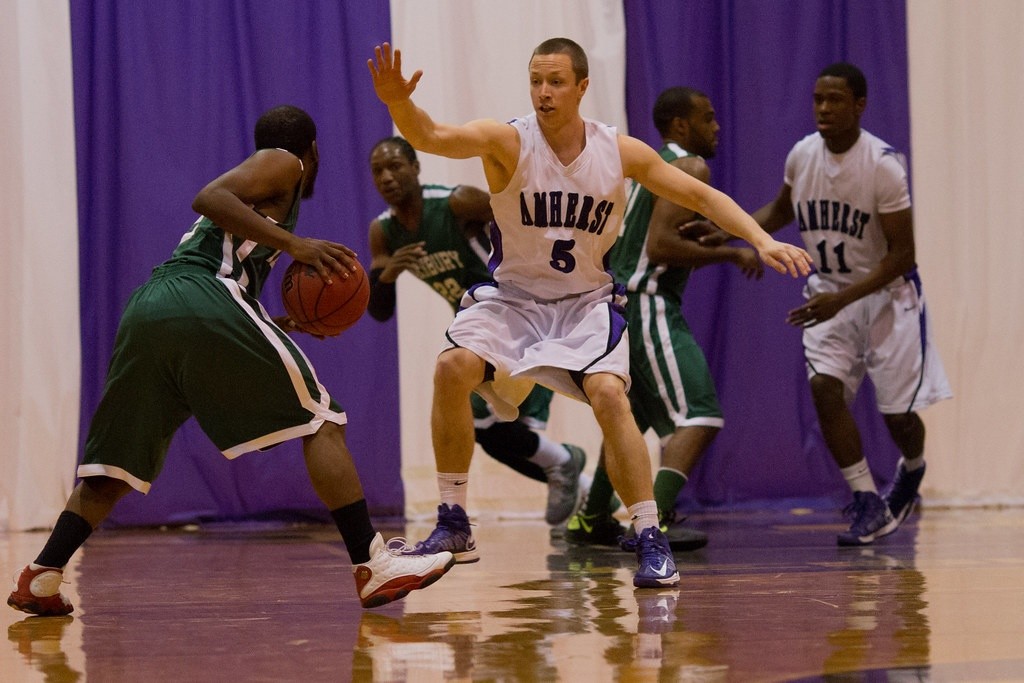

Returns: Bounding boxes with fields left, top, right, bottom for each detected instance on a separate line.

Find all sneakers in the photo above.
left=615, top=506, right=710, bottom=552
left=544, top=442, right=586, bottom=526
left=350, top=531, right=456, bottom=608
left=7, top=562, right=74, bottom=616
left=624, top=525, right=681, bottom=589
left=633, top=590, right=680, bottom=634
left=550, top=484, right=622, bottom=537
left=884, top=456, right=928, bottom=524
left=403, top=503, right=480, bottom=564
left=560, top=500, right=627, bottom=550
left=8, top=614, right=74, bottom=666
left=837, top=489, right=898, bottom=544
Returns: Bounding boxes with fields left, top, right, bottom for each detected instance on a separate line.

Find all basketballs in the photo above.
left=281, top=250, right=372, bottom=337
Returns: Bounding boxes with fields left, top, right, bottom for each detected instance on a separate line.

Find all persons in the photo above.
left=564, top=83, right=766, bottom=550
left=363, top=136, right=591, bottom=539
left=678, top=60, right=929, bottom=547
left=4, top=102, right=454, bottom=615
left=358, top=34, right=819, bottom=587
